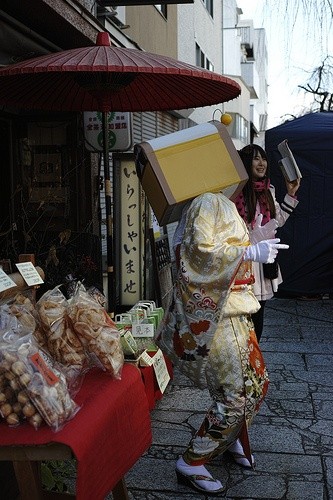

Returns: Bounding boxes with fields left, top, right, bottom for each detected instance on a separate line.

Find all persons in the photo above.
left=133, top=120, right=290, bottom=494
left=230, top=144, right=299, bottom=345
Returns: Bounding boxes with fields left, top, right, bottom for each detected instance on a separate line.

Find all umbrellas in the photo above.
left=0, top=31, right=242, bottom=322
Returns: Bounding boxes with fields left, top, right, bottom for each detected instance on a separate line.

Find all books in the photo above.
left=277, top=139, right=302, bottom=182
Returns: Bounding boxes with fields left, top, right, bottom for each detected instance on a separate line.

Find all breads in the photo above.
left=0, top=344, right=75, bottom=431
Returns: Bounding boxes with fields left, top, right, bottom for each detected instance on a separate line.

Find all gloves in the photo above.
left=248, top=214, right=279, bottom=245
left=243, top=239, right=289, bottom=264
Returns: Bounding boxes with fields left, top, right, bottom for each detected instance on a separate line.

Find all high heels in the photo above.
left=223, top=449, right=255, bottom=469
left=175, top=468, right=225, bottom=494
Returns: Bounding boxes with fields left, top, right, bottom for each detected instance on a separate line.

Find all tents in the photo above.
left=265, top=110, right=333, bottom=300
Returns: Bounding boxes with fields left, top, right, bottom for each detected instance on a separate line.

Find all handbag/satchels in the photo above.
left=262, top=263, right=278, bottom=279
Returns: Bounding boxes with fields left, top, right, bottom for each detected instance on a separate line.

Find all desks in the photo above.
left=0, top=349, right=174, bottom=500
